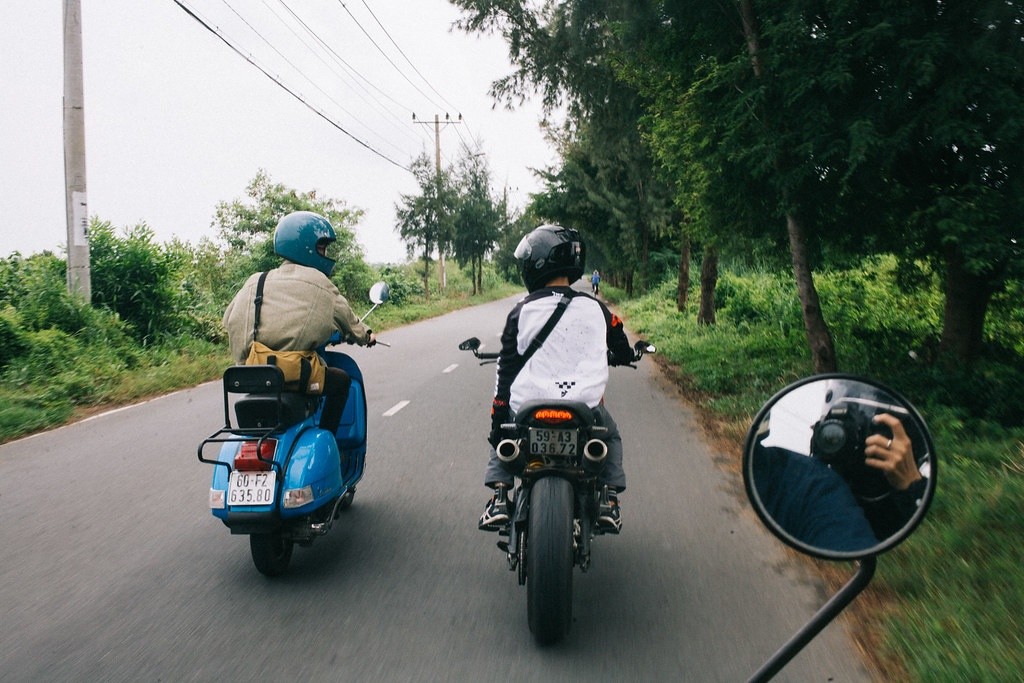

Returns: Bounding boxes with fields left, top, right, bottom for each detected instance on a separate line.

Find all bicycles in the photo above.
left=591, top=280, right=600, bottom=298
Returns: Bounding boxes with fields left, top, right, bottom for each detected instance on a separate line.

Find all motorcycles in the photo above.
left=458, top=337, right=658, bottom=646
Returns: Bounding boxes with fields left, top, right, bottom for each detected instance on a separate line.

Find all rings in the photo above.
left=886, top=440, right=892, bottom=448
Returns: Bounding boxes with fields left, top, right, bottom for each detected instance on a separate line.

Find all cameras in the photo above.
left=812, top=404, right=889, bottom=458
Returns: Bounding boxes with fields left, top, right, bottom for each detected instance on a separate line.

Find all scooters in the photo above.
left=197, top=279, right=392, bottom=577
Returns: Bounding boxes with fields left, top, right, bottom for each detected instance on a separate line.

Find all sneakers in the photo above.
left=477, top=502, right=511, bottom=533
left=597, top=504, right=624, bottom=535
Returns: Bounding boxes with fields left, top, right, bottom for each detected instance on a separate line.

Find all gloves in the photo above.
left=487, top=399, right=510, bottom=451
left=609, top=346, right=635, bottom=368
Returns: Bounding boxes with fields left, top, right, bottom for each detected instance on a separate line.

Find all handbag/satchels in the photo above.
left=245, top=340, right=330, bottom=397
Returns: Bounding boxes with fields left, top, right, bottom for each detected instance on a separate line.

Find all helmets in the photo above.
left=512, top=224, right=587, bottom=295
left=273, top=211, right=337, bottom=278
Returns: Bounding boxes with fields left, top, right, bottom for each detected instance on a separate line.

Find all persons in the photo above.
left=591, top=269, right=599, bottom=294
left=222, top=211, right=378, bottom=441
left=753, top=408, right=880, bottom=552
left=808, top=378, right=929, bottom=541
left=476, top=223, right=634, bottom=533
